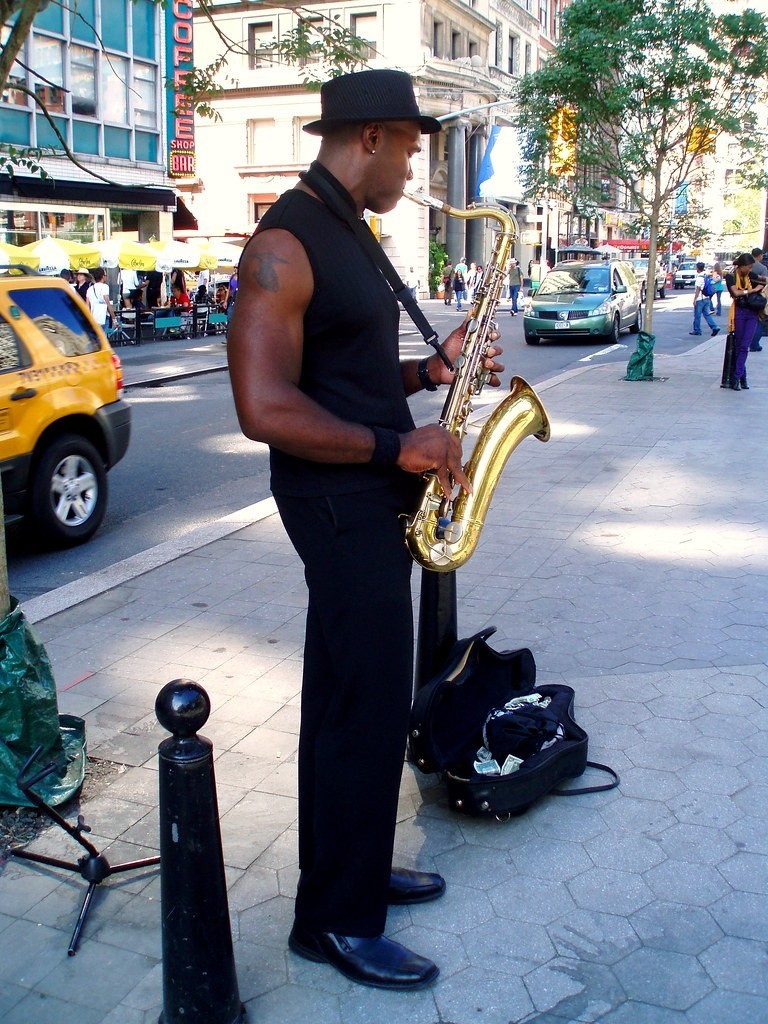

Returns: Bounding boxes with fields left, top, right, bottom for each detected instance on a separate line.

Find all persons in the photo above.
left=126, top=289, right=153, bottom=344
left=164, top=287, right=192, bottom=334
left=221, top=266, right=239, bottom=344
left=442, top=256, right=524, bottom=316
left=528, top=259, right=551, bottom=279
left=725, top=248, right=768, bottom=391
left=194, top=285, right=215, bottom=338
left=60, top=268, right=121, bottom=333
left=226, top=68, right=504, bottom=991
left=659, top=258, right=678, bottom=278
left=688, top=262, right=724, bottom=337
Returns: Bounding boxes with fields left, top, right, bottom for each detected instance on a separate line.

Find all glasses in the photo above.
left=234, top=266, right=238, bottom=269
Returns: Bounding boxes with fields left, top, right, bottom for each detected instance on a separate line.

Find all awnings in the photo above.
left=603, top=239, right=683, bottom=255
left=121, top=197, right=199, bottom=232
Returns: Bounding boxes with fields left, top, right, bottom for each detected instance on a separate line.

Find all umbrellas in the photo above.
left=0, top=235, right=245, bottom=309
left=557, top=243, right=623, bottom=260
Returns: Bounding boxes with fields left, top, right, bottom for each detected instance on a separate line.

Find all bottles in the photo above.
left=173, top=299, right=177, bottom=307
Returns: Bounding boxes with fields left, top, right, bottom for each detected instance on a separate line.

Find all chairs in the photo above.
left=111, top=303, right=216, bottom=346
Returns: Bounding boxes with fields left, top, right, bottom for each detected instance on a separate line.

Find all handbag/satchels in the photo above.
left=518, top=291, right=524, bottom=306
left=444, top=277, right=451, bottom=283
left=746, top=291, right=767, bottom=312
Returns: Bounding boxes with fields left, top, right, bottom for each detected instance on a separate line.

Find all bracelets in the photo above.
left=416, top=358, right=438, bottom=391
left=368, top=426, right=402, bottom=470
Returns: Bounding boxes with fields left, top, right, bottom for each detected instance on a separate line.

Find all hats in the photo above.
left=460, top=257, right=466, bottom=261
left=508, top=258, right=517, bottom=264
left=303, top=69, right=442, bottom=135
left=73, top=268, right=92, bottom=280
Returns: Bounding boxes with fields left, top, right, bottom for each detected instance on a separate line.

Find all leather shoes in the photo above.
left=288, top=923, right=439, bottom=990
left=388, top=866, right=447, bottom=906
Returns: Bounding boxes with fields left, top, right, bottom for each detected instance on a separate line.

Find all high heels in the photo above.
left=740, top=377, right=749, bottom=389
left=731, top=378, right=741, bottom=391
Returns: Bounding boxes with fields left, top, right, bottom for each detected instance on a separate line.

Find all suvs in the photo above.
left=0, top=264, right=131, bottom=549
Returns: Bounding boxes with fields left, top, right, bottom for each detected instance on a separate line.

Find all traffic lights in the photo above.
left=686, top=123, right=718, bottom=155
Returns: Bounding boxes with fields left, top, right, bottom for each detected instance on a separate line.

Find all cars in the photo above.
left=704, top=264, right=736, bottom=279
left=628, top=258, right=667, bottom=303
left=522, top=258, right=646, bottom=344
left=673, top=262, right=698, bottom=290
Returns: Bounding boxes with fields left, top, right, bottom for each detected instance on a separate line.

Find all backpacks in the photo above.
left=699, top=275, right=715, bottom=296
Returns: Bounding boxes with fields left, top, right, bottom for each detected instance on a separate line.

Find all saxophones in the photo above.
left=404, top=189, right=550, bottom=572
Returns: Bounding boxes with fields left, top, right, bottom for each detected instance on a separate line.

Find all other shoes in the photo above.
left=511, top=310, right=516, bottom=316
left=709, top=311, right=715, bottom=314
left=463, top=290, right=467, bottom=300
left=749, top=346, right=762, bottom=352
left=711, top=329, right=720, bottom=336
left=456, top=307, right=459, bottom=311
left=221, top=340, right=227, bottom=344
left=689, top=331, right=701, bottom=335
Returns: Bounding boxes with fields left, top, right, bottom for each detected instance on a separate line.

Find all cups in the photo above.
left=157, top=298, right=161, bottom=307
